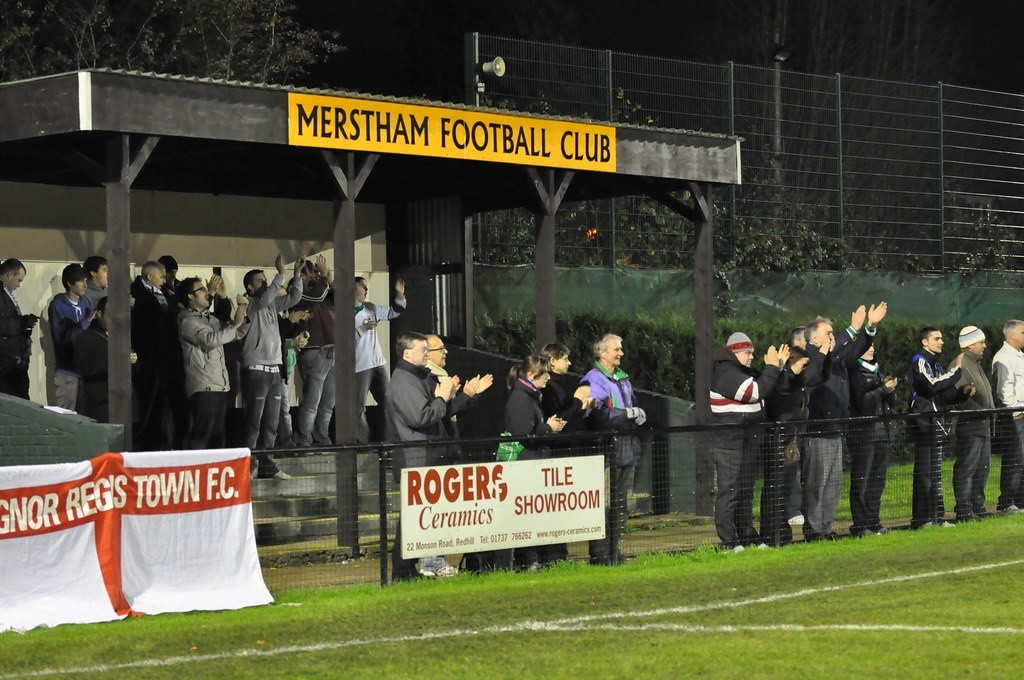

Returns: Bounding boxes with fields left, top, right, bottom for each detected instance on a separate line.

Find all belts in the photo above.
left=248, top=364, right=279, bottom=373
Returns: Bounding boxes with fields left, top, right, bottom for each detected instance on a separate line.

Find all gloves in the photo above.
left=635, top=409, right=647, bottom=425
left=626, top=407, right=640, bottom=419
left=23, top=315, right=39, bottom=328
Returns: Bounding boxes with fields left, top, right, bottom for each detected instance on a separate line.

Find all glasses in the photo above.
left=428, top=346, right=444, bottom=352
left=192, top=286, right=206, bottom=294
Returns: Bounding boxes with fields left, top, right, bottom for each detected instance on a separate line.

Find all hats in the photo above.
left=726, top=332, right=755, bottom=354
left=958, top=325, right=985, bottom=348
left=159, top=255, right=179, bottom=274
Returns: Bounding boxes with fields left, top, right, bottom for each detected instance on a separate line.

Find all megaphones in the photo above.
left=474, top=57, right=505, bottom=77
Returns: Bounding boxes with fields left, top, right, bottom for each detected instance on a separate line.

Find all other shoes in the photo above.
left=392, top=503, right=1024, bottom=575
left=251, top=461, right=291, bottom=480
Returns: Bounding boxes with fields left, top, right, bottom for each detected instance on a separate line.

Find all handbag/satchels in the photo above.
left=496, top=433, right=524, bottom=462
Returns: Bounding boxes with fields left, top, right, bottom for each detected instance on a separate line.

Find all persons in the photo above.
left=908, top=319, right=1024, bottom=530
left=711, top=301, right=898, bottom=555
left=49, top=256, right=405, bottom=478
left=385, top=330, right=494, bottom=585
left=0, top=259, right=39, bottom=400
left=498, top=332, right=648, bottom=576
